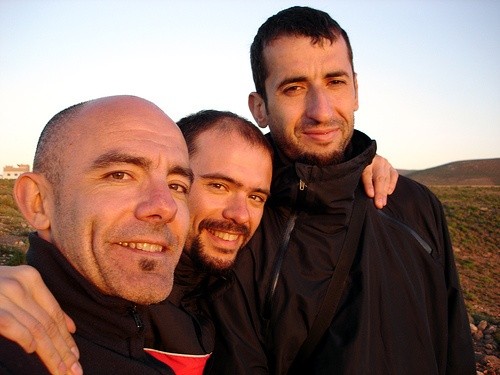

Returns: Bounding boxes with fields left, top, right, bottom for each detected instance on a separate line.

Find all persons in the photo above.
left=0, top=95, right=195, bottom=375
left=0, top=110, right=398, bottom=375
left=235, top=6, right=476, bottom=374
